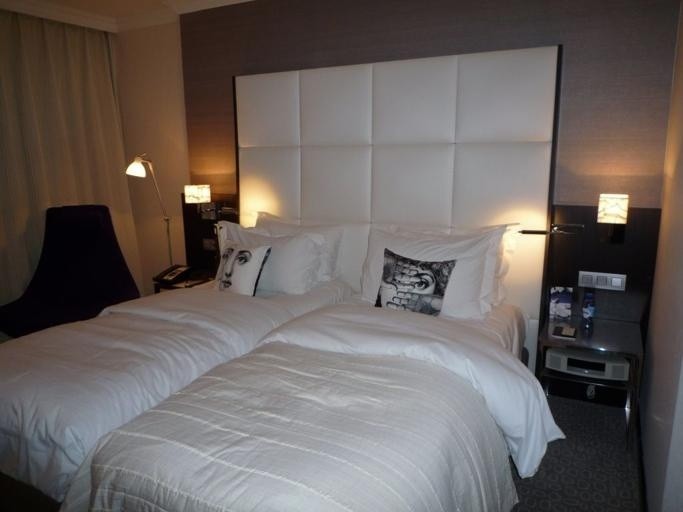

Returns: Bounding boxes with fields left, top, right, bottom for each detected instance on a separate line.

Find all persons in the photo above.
left=221, top=247, right=252, bottom=289
left=377, top=248, right=447, bottom=316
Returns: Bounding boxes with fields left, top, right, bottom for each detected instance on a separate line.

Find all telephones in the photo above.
left=152, top=264, right=196, bottom=284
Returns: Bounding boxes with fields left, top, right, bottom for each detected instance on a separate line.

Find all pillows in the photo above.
left=360, top=224, right=492, bottom=320
left=481, top=225, right=507, bottom=311
left=215, top=221, right=306, bottom=296
left=247, top=225, right=344, bottom=283
left=214, top=245, right=272, bottom=296
left=374, top=247, right=458, bottom=317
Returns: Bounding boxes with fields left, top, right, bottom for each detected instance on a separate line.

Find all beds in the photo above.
left=49, top=291, right=570, bottom=511
left=0, top=272, right=360, bottom=509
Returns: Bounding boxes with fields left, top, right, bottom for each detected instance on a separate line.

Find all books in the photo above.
left=551, top=326, right=578, bottom=341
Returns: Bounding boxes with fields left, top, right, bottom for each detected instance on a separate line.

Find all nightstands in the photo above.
left=530, top=310, right=645, bottom=454
left=152, top=268, right=215, bottom=294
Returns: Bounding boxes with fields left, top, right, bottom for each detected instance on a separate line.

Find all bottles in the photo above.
left=580, top=287, right=596, bottom=339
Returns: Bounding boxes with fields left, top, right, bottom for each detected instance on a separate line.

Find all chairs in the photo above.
left=0, top=203, right=142, bottom=342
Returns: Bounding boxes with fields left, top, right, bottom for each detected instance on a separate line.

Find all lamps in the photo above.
left=514, top=220, right=587, bottom=272
left=594, top=190, right=632, bottom=243
left=180, top=183, right=222, bottom=223
left=122, top=151, right=178, bottom=270
